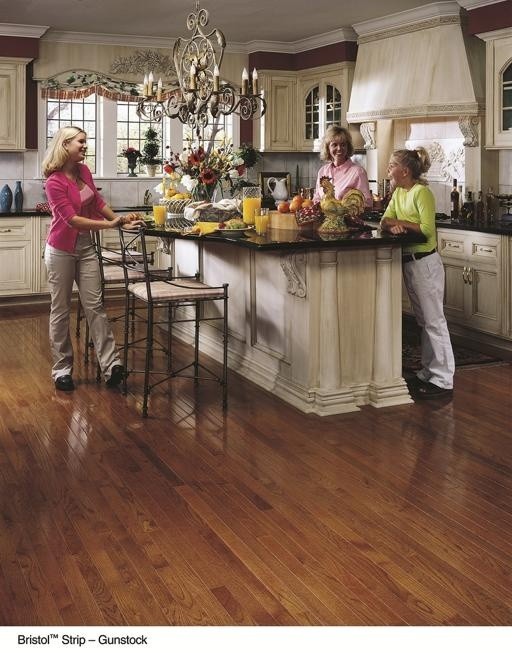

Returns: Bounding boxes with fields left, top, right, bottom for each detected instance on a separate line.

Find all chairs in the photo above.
left=76, top=223, right=228, bottom=417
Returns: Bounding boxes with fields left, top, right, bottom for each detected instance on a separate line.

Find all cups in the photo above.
left=254, top=208, right=269, bottom=237
left=242, top=186, right=262, bottom=223
left=152, top=200, right=168, bottom=226
left=267, top=175, right=289, bottom=207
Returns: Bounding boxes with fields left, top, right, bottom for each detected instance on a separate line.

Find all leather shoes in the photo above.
left=406, top=375, right=424, bottom=387
left=55, top=375, right=74, bottom=390
left=107, top=367, right=128, bottom=389
left=418, top=382, right=454, bottom=399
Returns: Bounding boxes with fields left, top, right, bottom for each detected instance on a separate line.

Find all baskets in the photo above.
left=158, top=197, right=193, bottom=214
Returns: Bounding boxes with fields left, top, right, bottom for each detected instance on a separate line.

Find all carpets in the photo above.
left=402, top=313, right=511, bottom=375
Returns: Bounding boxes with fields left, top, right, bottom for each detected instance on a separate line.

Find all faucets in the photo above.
left=144, top=189, right=151, bottom=204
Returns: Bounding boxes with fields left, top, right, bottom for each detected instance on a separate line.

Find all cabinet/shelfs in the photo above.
left=474, top=27, right=512, bottom=151
left=436, top=227, right=511, bottom=342
left=402, top=276, right=416, bottom=317
left=0, top=55, right=34, bottom=153
left=299, top=60, right=368, bottom=154
left=36, top=215, right=101, bottom=292
left=251, top=70, right=299, bottom=153
left=0, top=216, right=36, bottom=298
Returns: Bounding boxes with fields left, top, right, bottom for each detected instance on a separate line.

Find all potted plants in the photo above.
left=136, top=126, right=162, bottom=177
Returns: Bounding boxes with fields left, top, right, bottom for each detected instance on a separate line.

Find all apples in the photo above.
left=277, top=194, right=314, bottom=212
left=165, top=189, right=191, bottom=200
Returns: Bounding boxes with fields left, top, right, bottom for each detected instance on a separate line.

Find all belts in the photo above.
left=402, top=248, right=437, bottom=262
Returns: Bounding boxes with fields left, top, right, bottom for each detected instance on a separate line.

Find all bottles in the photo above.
left=451, top=177, right=496, bottom=224
left=14, top=180, right=24, bottom=211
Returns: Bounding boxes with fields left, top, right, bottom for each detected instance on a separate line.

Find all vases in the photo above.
left=14, top=181, right=23, bottom=213
left=128, top=157, right=137, bottom=177
left=192, top=181, right=217, bottom=202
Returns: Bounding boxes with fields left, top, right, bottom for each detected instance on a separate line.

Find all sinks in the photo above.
left=123, top=206, right=153, bottom=210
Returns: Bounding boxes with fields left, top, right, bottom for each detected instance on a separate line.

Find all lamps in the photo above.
left=136, top=0, right=267, bottom=129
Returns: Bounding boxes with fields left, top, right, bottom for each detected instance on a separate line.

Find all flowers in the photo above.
left=159, top=121, right=263, bottom=200
left=120, top=148, right=142, bottom=158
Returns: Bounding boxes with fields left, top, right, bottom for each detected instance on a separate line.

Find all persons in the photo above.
left=42, top=125, right=147, bottom=389
left=312, top=125, right=374, bottom=211
left=379, top=145, right=454, bottom=394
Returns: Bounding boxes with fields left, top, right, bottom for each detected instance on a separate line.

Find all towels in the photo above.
left=183, top=197, right=242, bottom=222
left=294, top=201, right=323, bottom=226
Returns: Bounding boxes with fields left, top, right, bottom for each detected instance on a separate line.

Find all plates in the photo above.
left=216, top=225, right=252, bottom=236
left=142, top=219, right=152, bottom=223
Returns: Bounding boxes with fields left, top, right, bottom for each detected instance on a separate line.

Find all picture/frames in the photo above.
left=256, top=171, right=291, bottom=197
